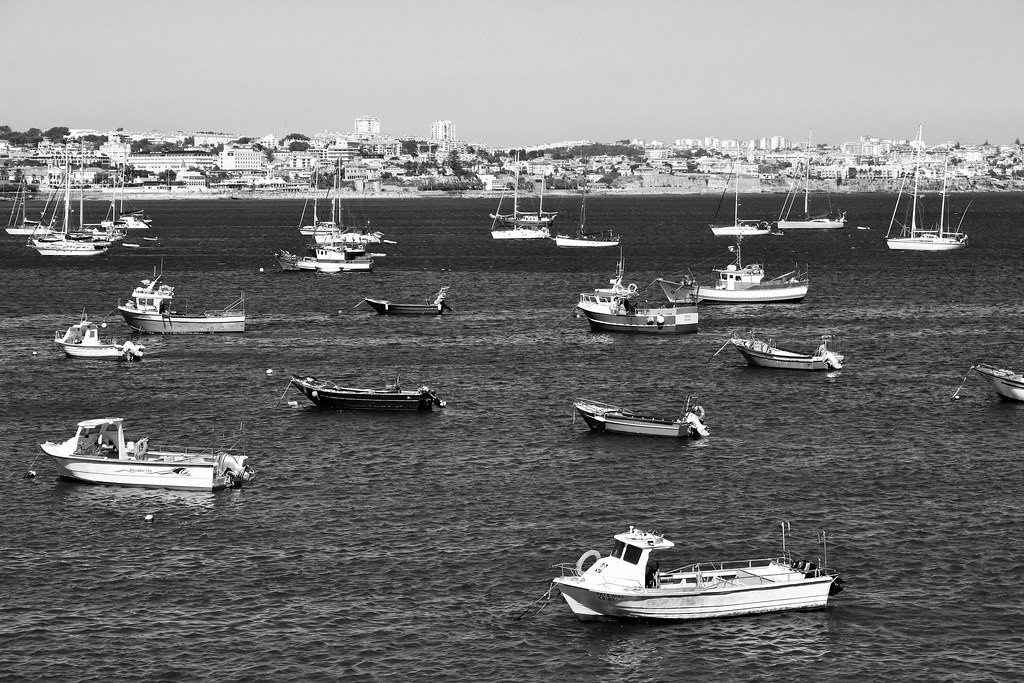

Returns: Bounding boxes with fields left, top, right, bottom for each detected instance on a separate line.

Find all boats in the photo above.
left=552, top=521, right=846, bottom=625
left=729, top=329, right=845, bottom=371
left=55, top=305, right=144, bottom=361
left=655, top=232, right=810, bottom=304
left=118, top=274, right=246, bottom=335
left=40, top=417, right=256, bottom=491
left=576, top=246, right=700, bottom=335
left=290, top=372, right=446, bottom=415
left=364, top=285, right=450, bottom=315
left=972, top=358, right=1024, bottom=403
left=574, top=392, right=708, bottom=438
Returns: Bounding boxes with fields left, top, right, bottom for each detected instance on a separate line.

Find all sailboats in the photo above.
left=489, top=150, right=558, bottom=240
left=709, top=146, right=772, bottom=236
left=883, top=123, right=969, bottom=251
left=555, top=153, right=621, bottom=249
left=5, top=139, right=153, bottom=256
left=271, top=154, right=385, bottom=273
left=777, top=131, right=847, bottom=230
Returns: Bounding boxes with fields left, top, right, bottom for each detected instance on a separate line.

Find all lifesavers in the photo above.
left=628, top=283, right=637, bottom=291
left=616, top=285, right=623, bottom=295
left=134, top=440, right=148, bottom=456
left=694, top=405, right=706, bottom=420
left=576, top=550, right=602, bottom=577
